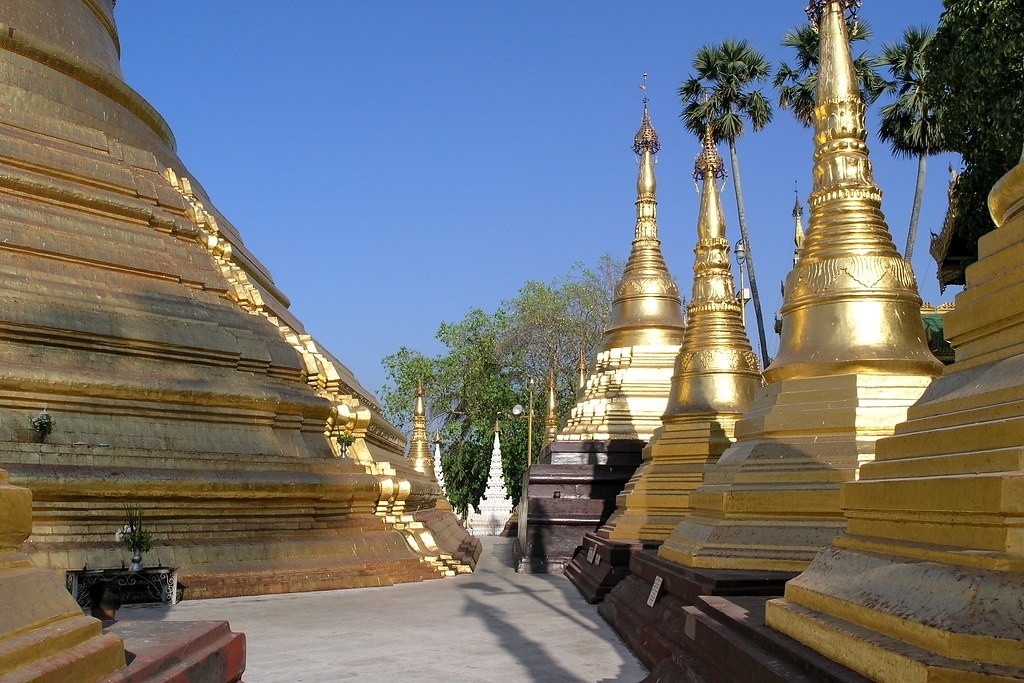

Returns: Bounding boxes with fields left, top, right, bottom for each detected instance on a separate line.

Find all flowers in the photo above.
left=114, top=498, right=154, bottom=556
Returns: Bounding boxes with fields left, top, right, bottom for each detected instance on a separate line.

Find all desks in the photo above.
left=65, top=566, right=179, bottom=607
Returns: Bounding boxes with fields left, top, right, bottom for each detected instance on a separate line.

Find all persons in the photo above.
left=89, top=578, right=122, bottom=621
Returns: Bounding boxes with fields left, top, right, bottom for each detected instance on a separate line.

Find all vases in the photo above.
left=127, top=555, right=144, bottom=571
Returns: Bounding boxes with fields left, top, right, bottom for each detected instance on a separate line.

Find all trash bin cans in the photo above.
left=87, top=581, right=122, bottom=620
